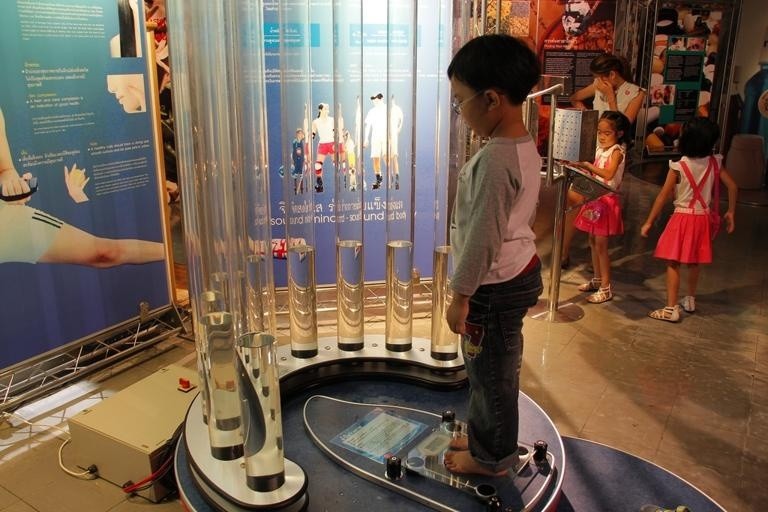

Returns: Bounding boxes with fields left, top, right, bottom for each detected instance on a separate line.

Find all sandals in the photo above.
left=578, top=277, right=602, bottom=292
left=678, top=296, right=695, bottom=313
left=584, top=283, right=612, bottom=304
left=647, top=304, right=681, bottom=322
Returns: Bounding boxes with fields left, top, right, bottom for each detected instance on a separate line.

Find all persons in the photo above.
left=641, top=113, right=739, bottom=323
left=63, top=162, right=91, bottom=203
left=107, top=75, right=147, bottom=113
left=442, top=34, right=545, bottom=477
left=560, top=54, right=649, bottom=274
left=289, top=92, right=405, bottom=193
left=568, top=108, right=633, bottom=304
left=0, top=104, right=165, bottom=269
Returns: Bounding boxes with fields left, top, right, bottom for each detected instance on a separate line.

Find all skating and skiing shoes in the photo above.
left=314, top=177, right=322, bottom=192
left=372, top=174, right=382, bottom=188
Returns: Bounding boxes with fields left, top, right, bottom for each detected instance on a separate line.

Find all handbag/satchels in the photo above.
left=709, top=207, right=725, bottom=240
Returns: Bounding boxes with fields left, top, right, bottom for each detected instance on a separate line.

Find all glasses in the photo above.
left=451, top=87, right=505, bottom=115
